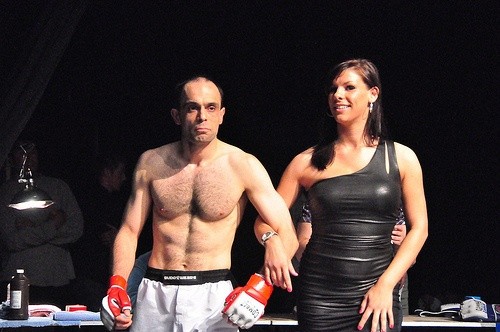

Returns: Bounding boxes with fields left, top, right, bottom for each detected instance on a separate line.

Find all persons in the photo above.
left=254, top=60, right=429, bottom=332
left=127, top=249, right=239, bottom=312
left=296, top=188, right=410, bottom=317
left=1, top=132, right=85, bottom=309
left=101, top=77, right=301, bottom=332
left=73, top=151, right=127, bottom=290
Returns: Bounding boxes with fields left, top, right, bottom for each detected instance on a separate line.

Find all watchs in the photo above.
left=261, top=231, right=280, bottom=247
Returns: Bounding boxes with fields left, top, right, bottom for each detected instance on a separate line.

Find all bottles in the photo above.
left=9, top=269, right=30, bottom=320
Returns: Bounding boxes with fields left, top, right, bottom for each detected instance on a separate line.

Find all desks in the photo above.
left=1, top=310, right=496, bottom=330
